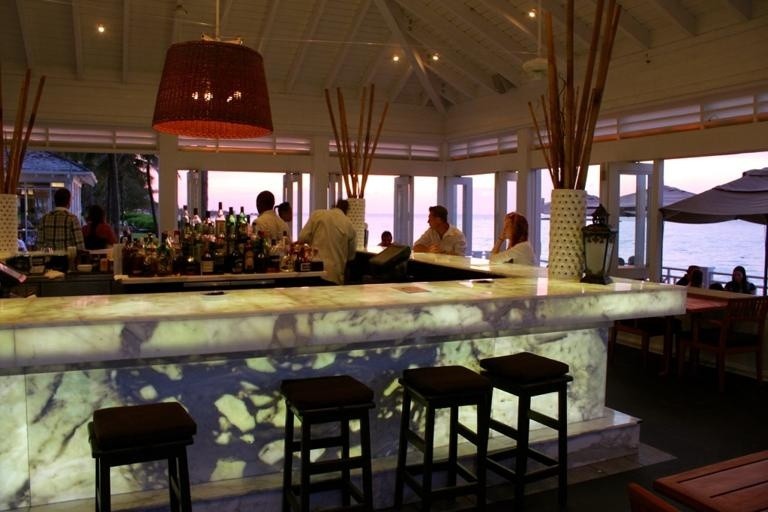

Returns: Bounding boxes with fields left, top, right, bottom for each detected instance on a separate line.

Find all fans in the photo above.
left=521, top=1, right=551, bottom=80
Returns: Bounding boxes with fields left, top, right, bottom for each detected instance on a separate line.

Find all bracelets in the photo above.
left=498, top=237, right=504, bottom=242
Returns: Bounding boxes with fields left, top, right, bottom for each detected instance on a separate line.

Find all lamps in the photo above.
left=150, top=0, right=273, bottom=139
left=579, top=204, right=617, bottom=285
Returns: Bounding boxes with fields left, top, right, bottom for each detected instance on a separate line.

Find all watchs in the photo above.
left=376, top=231, right=393, bottom=246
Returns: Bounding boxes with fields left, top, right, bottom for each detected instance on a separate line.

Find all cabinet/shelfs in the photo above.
left=0, top=246, right=688, bottom=511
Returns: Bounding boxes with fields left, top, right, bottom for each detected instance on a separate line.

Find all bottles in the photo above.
left=231, top=229, right=243, bottom=274
left=237, top=206, right=247, bottom=243
left=125, top=233, right=133, bottom=275
left=243, top=236, right=255, bottom=272
left=266, top=229, right=273, bottom=253
left=173, top=230, right=184, bottom=274
left=201, top=238, right=214, bottom=274
left=252, top=223, right=259, bottom=239
left=280, top=249, right=294, bottom=272
left=132, top=238, right=143, bottom=273
left=258, top=240, right=266, bottom=272
left=192, top=208, right=201, bottom=226
left=205, top=209, right=213, bottom=233
left=227, top=207, right=235, bottom=250
left=215, top=238, right=226, bottom=273
left=144, top=233, right=158, bottom=273
left=248, top=215, right=252, bottom=234
left=157, top=233, right=175, bottom=273
left=281, top=231, right=289, bottom=251
left=180, top=205, right=191, bottom=232
left=270, top=239, right=281, bottom=269
left=215, top=202, right=225, bottom=242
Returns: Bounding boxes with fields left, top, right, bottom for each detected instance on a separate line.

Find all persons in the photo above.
left=618, top=257, right=625, bottom=266
left=296, top=200, right=359, bottom=286
left=413, top=205, right=466, bottom=256
left=628, top=256, right=645, bottom=282
left=34, top=188, right=86, bottom=256
left=725, top=266, right=756, bottom=294
left=489, top=213, right=537, bottom=265
left=687, top=270, right=703, bottom=288
left=676, top=265, right=700, bottom=288
left=80, top=204, right=119, bottom=250
left=255, top=191, right=291, bottom=250
left=274, top=202, right=293, bottom=223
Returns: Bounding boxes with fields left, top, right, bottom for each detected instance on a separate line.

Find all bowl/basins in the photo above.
left=77, top=264, right=93, bottom=272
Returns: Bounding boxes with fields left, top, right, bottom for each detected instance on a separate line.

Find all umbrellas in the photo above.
left=618, top=184, right=698, bottom=221
left=657, top=166, right=768, bottom=298
left=539, top=195, right=601, bottom=215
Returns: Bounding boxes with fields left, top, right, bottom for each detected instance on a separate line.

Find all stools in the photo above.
left=87, top=402, right=197, bottom=512
left=393, top=366, right=493, bottom=512
left=476, top=353, right=573, bottom=509
left=280, top=375, right=375, bottom=512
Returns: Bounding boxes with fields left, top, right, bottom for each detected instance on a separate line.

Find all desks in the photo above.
left=655, top=450, right=768, bottom=512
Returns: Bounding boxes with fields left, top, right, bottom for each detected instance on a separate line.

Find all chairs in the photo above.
left=629, top=481, right=677, bottom=512
left=677, top=296, right=768, bottom=401
left=609, top=316, right=675, bottom=373
